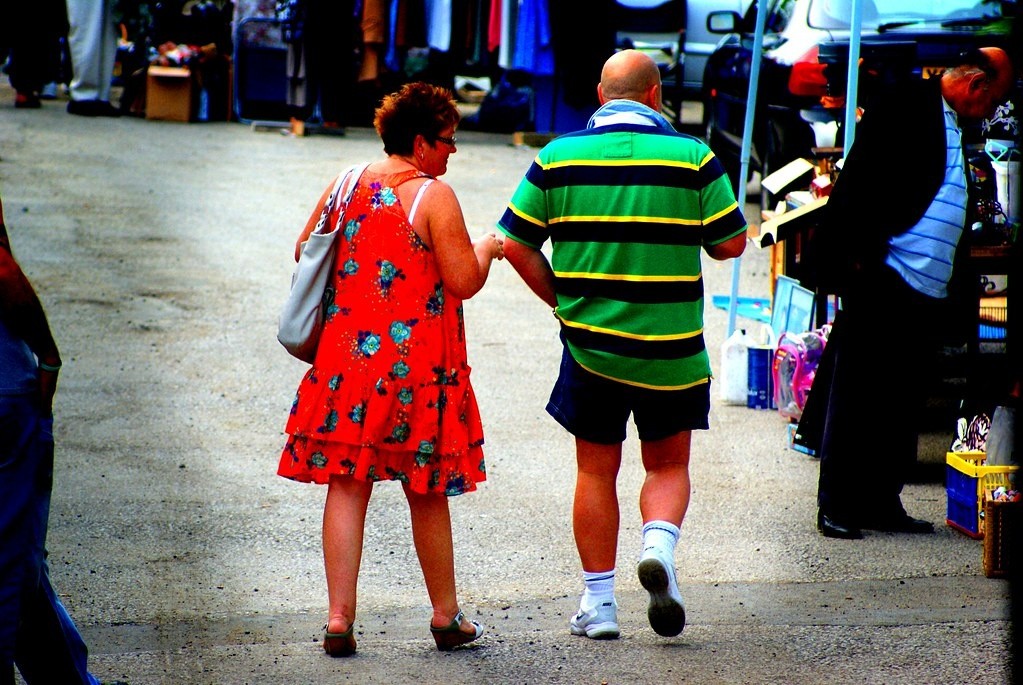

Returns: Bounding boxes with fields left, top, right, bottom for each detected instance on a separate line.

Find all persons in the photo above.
left=0, top=0, right=127, bottom=118
left=0, top=204, right=100, bottom=685
left=289, top=0, right=360, bottom=136
left=123, top=0, right=240, bottom=124
left=498, top=48, right=749, bottom=640
left=293, top=82, right=507, bottom=659
left=791, top=45, right=1012, bottom=538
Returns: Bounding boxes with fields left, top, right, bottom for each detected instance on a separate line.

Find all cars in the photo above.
left=702, top=0, right=1023, bottom=219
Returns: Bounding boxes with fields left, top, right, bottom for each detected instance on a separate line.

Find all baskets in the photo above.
left=980, top=489, right=1023, bottom=578
left=946, top=451, right=1023, bottom=538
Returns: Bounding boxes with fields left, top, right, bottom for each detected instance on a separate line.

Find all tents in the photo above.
left=730, top=1, right=1023, bottom=338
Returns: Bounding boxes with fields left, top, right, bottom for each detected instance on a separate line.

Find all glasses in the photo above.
left=436, top=135, right=458, bottom=147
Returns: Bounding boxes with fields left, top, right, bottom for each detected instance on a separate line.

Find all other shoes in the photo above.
left=41, top=82, right=57, bottom=97
left=15, top=91, right=40, bottom=108
left=67, top=100, right=123, bottom=117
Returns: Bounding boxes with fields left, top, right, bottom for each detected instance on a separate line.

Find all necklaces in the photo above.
left=389, top=157, right=409, bottom=163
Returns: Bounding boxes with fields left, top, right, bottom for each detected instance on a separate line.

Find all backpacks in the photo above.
left=772, top=322, right=832, bottom=422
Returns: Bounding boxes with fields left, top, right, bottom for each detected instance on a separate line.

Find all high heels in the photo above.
left=430, top=608, right=484, bottom=652
left=320, top=621, right=356, bottom=658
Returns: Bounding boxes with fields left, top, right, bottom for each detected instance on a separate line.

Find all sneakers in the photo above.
left=637, top=546, right=685, bottom=637
left=571, top=595, right=620, bottom=638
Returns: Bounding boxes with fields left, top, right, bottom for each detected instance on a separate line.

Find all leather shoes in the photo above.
left=818, top=507, right=863, bottom=539
left=887, top=515, right=934, bottom=533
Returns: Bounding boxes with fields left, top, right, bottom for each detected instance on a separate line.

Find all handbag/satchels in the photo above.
left=277, top=162, right=371, bottom=365
left=721, top=317, right=774, bottom=404
